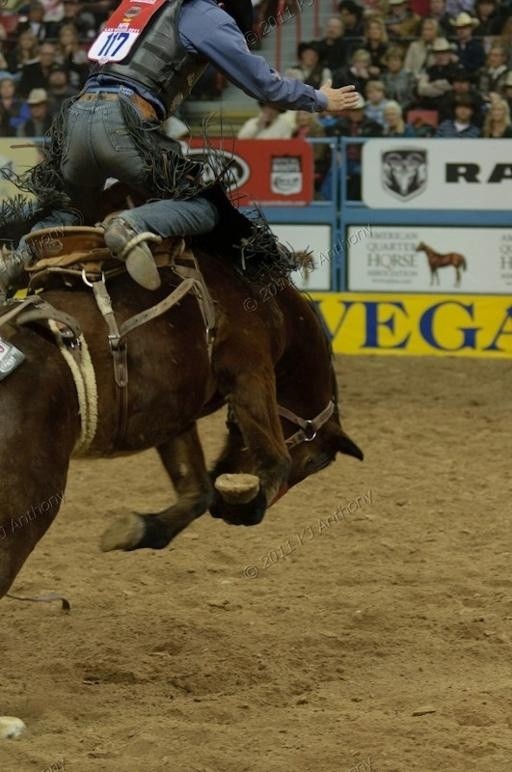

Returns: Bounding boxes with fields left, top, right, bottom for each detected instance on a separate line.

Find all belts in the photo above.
left=76, top=92, right=119, bottom=103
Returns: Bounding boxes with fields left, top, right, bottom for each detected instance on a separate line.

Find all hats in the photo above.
left=448, top=12, right=480, bottom=29
left=27, top=88, right=48, bottom=105
left=428, top=37, right=458, bottom=52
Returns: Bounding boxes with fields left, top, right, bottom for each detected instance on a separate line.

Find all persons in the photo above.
left=2, top=2, right=512, bottom=201
left=1, top=0, right=358, bottom=291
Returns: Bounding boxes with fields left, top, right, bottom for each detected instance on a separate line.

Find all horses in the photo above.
left=415, top=241, right=466, bottom=287
left=1, top=188, right=364, bottom=605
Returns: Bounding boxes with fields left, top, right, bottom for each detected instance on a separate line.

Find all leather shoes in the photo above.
left=104, top=218, right=163, bottom=291
